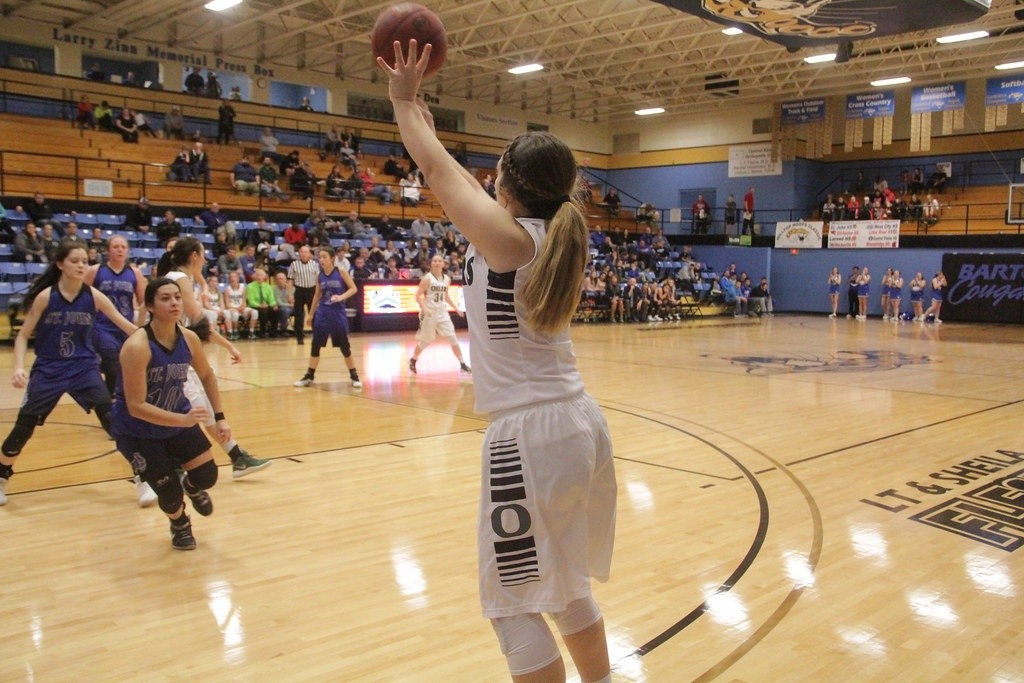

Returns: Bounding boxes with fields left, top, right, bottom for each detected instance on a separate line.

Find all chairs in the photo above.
left=0, top=210, right=725, bottom=332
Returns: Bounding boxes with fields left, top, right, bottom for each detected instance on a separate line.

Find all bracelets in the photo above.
left=214, top=412, right=225, bottom=422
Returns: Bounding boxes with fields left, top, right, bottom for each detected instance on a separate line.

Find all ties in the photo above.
left=259, top=286, right=263, bottom=304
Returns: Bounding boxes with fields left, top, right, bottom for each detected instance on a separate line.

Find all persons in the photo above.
left=823, top=165, right=952, bottom=228
left=85, top=233, right=149, bottom=405
left=0, top=190, right=473, bottom=345
left=75, top=58, right=499, bottom=206
left=294, top=246, right=363, bottom=389
left=846, top=265, right=871, bottom=320
left=579, top=170, right=775, bottom=323
left=157, top=237, right=272, bottom=479
left=880, top=266, right=904, bottom=321
left=406, top=254, right=471, bottom=376
left=908, top=271, right=926, bottom=321
left=918, top=272, right=948, bottom=323
left=112, top=277, right=231, bottom=551
left=826, top=266, right=842, bottom=318
left=376, top=38, right=618, bottom=682
left=0, top=236, right=160, bottom=507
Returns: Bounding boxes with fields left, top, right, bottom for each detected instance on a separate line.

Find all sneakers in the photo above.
left=409, top=362, right=417, bottom=373
left=170, top=520, right=195, bottom=550
left=181, top=479, right=212, bottom=516
left=351, top=376, right=362, bottom=388
left=231, top=452, right=271, bottom=478
left=294, top=375, right=313, bottom=386
left=461, top=366, right=471, bottom=373
left=134, top=475, right=158, bottom=506
left=0, top=478, right=9, bottom=504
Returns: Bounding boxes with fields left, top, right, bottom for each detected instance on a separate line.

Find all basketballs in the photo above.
left=371, top=2, right=449, bottom=81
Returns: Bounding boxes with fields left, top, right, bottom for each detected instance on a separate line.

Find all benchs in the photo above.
left=0, top=93, right=660, bottom=242
left=802, top=186, right=1024, bottom=234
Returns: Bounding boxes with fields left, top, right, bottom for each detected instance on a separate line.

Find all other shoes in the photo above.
left=610, top=313, right=694, bottom=323
left=227, top=331, right=290, bottom=340
left=829, top=313, right=942, bottom=323
